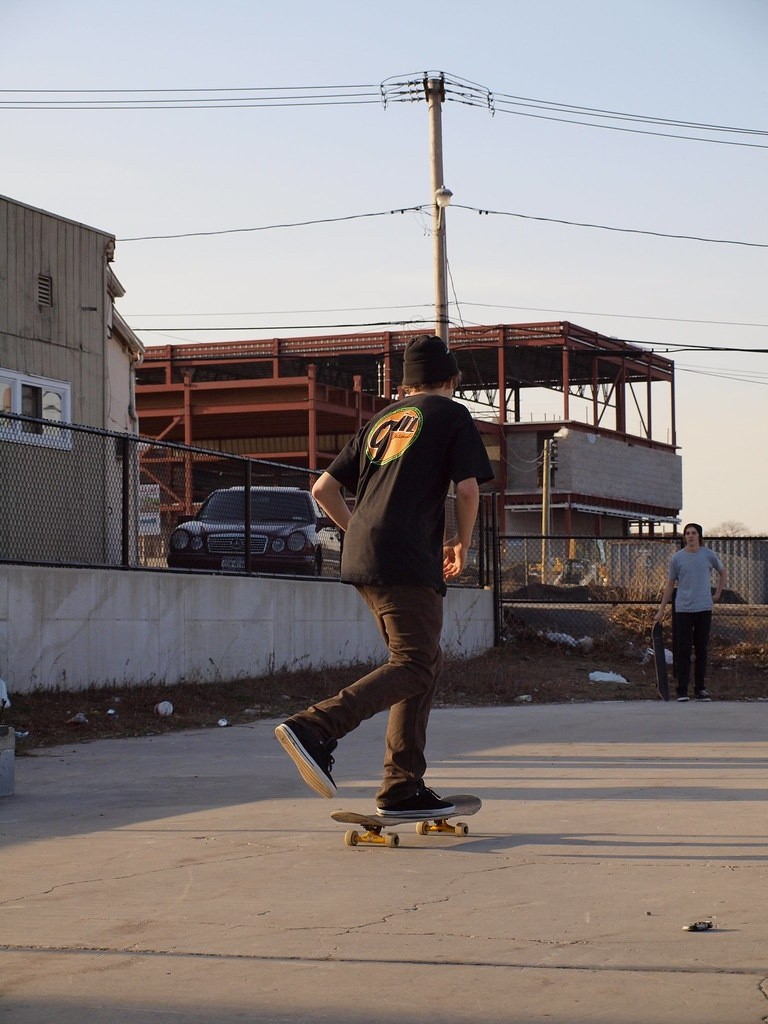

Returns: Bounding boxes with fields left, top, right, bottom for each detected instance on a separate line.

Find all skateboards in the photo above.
left=333, top=794, right=482, bottom=845
left=650, top=619, right=673, bottom=700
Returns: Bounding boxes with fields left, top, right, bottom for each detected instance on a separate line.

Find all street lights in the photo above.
left=430, top=186, right=454, bottom=355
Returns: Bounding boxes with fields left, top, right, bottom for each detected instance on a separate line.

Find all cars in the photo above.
left=167, top=486, right=336, bottom=576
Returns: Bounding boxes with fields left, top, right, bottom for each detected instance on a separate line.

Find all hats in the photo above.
left=402, top=335, right=458, bottom=385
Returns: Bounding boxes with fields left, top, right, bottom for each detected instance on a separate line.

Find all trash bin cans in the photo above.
left=560, top=556, right=590, bottom=602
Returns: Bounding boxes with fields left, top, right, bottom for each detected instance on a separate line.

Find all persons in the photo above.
left=275, top=336, right=495, bottom=818
left=653, top=523, right=727, bottom=702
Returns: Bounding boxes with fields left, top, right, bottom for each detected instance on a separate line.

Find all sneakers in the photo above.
left=275, top=719, right=338, bottom=798
left=677, top=693, right=690, bottom=701
left=376, top=778, right=455, bottom=817
left=696, top=689, right=711, bottom=702
